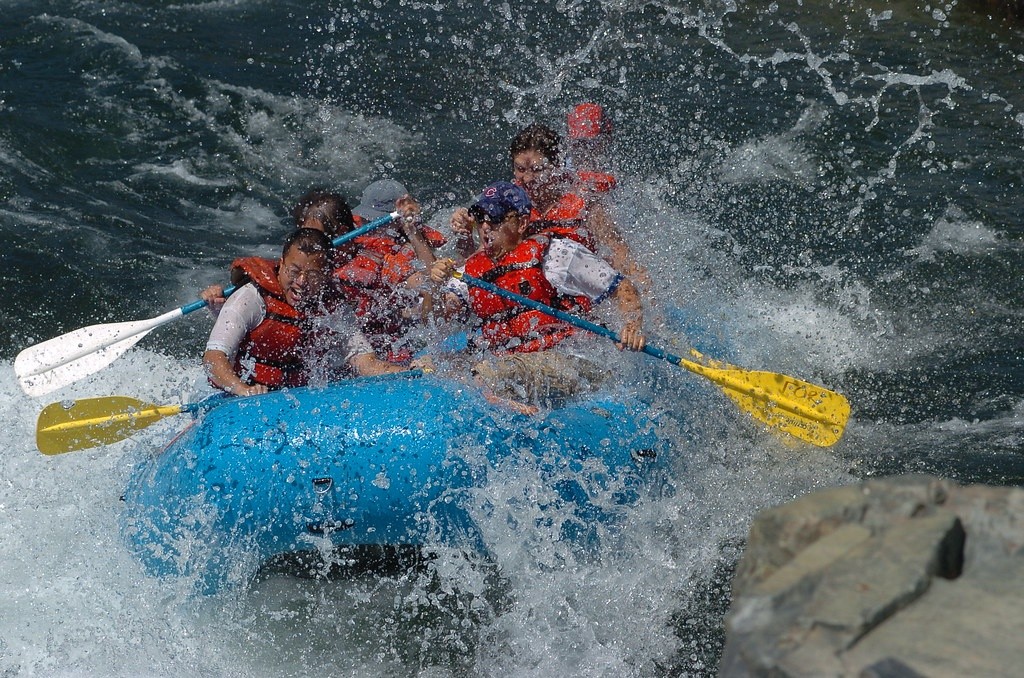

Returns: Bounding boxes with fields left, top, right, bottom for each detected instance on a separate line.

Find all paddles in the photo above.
left=12, top=195, right=412, bottom=398
left=37, top=363, right=427, bottom=457
left=435, top=263, right=850, bottom=449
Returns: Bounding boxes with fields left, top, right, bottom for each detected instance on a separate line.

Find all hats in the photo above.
left=468, top=182, right=532, bottom=217
left=474, top=212, right=518, bottom=223
left=566, top=104, right=612, bottom=139
left=351, top=179, right=408, bottom=223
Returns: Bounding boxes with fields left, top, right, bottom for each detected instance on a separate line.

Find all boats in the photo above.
left=126, top=361, right=693, bottom=603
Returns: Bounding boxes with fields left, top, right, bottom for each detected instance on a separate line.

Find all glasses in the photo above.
left=284, top=264, right=318, bottom=284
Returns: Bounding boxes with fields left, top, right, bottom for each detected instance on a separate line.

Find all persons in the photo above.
left=204, top=101, right=650, bottom=390
left=204, top=226, right=411, bottom=396
